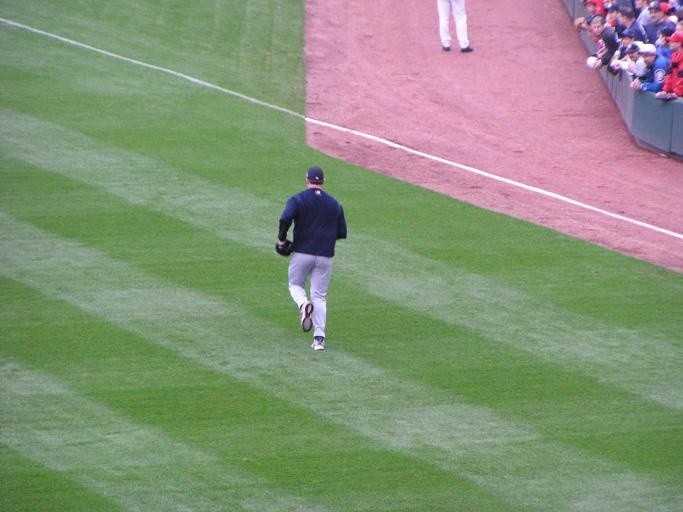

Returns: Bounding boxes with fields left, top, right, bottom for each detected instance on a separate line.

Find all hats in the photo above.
left=624, top=43, right=640, bottom=54
left=602, top=1, right=621, bottom=12
left=657, top=26, right=675, bottom=36
left=665, top=31, right=683, bottom=42
left=648, top=2, right=661, bottom=11
left=307, top=167, right=325, bottom=184
left=638, top=43, right=657, bottom=55
left=619, top=6, right=635, bottom=18
left=619, top=29, right=635, bottom=38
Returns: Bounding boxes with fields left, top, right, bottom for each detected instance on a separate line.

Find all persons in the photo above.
left=275, top=166, right=347, bottom=351
left=574, top=0, right=683, bottom=101
left=437, top=1, right=474, bottom=52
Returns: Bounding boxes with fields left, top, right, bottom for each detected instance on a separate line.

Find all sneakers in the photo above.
left=309, top=337, right=326, bottom=350
left=461, top=45, right=474, bottom=52
left=301, top=301, right=313, bottom=332
left=443, top=46, right=452, bottom=51
